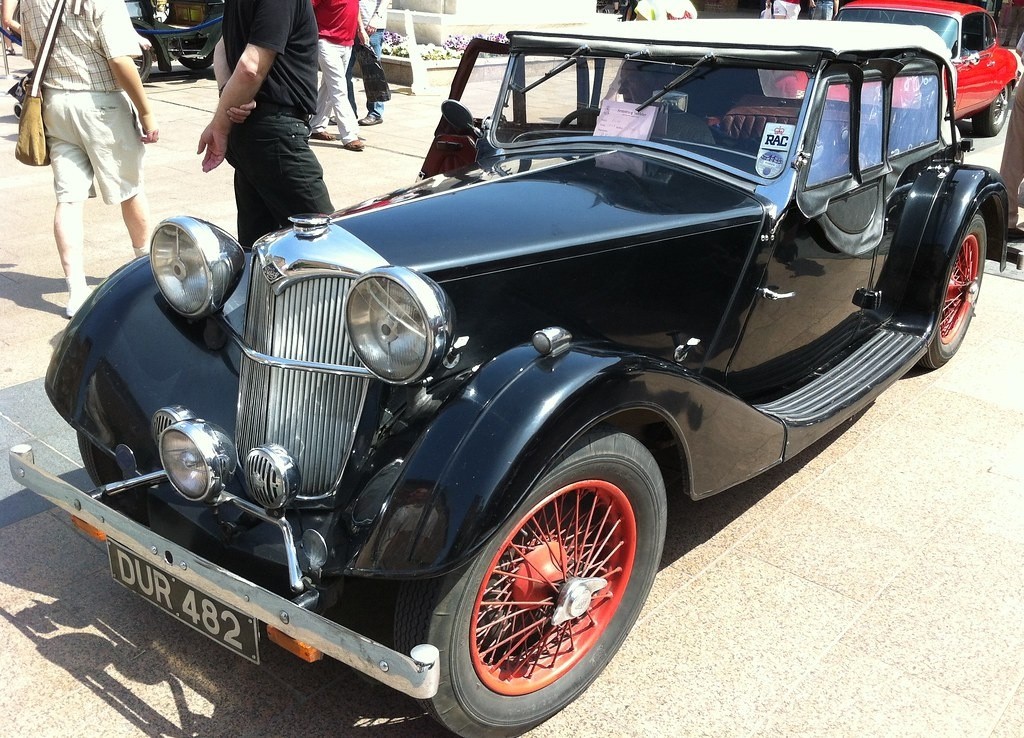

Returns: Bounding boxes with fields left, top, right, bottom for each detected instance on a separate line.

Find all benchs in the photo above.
left=721, top=98, right=896, bottom=170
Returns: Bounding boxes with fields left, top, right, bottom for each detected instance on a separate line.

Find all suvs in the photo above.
left=10, top=19, right=1024, bottom=738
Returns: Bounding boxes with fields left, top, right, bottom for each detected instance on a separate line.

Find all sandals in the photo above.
left=6, top=49, right=16, bottom=55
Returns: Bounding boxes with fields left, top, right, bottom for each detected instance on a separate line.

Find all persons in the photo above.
left=2, top=0, right=22, bottom=56
left=618, top=60, right=716, bottom=147
left=998, top=32, right=1024, bottom=239
left=326, top=0, right=388, bottom=125
left=198, top=0, right=335, bottom=253
left=809, top=0, right=839, bottom=20
left=599, top=0, right=698, bottom=109
left=309, top=0, right=365, bottom=151
left=765, top=0, right=802, bottom=20
left=20, top=0, right=175, bottom=320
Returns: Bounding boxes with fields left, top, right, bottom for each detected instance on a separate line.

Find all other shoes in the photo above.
left=1008, top=228, right=1024, bottom=238
left=66, top=292, right=94, bottom=317
left=1002, top=41, right=1009, bottom=46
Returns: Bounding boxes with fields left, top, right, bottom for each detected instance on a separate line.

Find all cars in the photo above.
left=768, top=0, right=1019, bottom=137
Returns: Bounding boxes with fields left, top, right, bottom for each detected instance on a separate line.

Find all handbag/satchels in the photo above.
left=7, top=69, right=50, bottom=167
left=356, top=44, right=391, bottom=102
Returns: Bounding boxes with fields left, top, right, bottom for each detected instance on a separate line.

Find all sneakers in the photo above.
left=329, top=116, right=337, bottom=125
left=310, top=130, right=335, bottom=140
left=358, top=116, right=383, bottom=125
left=346, top=139, right=365, bottom=150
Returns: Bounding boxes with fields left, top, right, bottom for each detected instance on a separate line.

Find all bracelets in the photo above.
left=357, top=28, right=363, bottom=32
